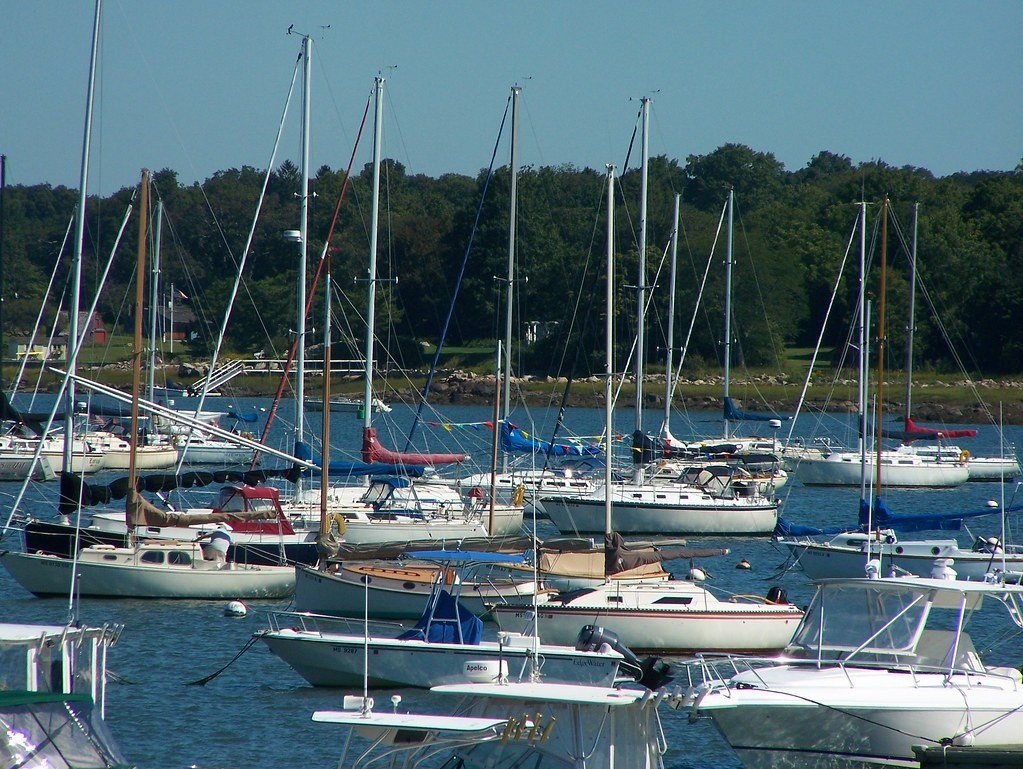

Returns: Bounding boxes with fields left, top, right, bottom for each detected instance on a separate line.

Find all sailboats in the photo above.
left=0, top=3, right=1023, bottom=768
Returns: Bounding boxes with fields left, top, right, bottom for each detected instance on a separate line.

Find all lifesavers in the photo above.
left=172, top=435, right=177, bottom=441
left=325, top=512, right=346, bottom=536
left=514, top=484, right=525, bottom=505
left=241, top=432, right=252, bottom=444
left=960, top=450, right=970, bottom=463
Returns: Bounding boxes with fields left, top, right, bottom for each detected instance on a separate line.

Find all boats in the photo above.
left=675, top=569, right=1022, bottom=769
left=305, top=398, right=384, bottom=414
left=146, top=384, right=195, bottom=398
